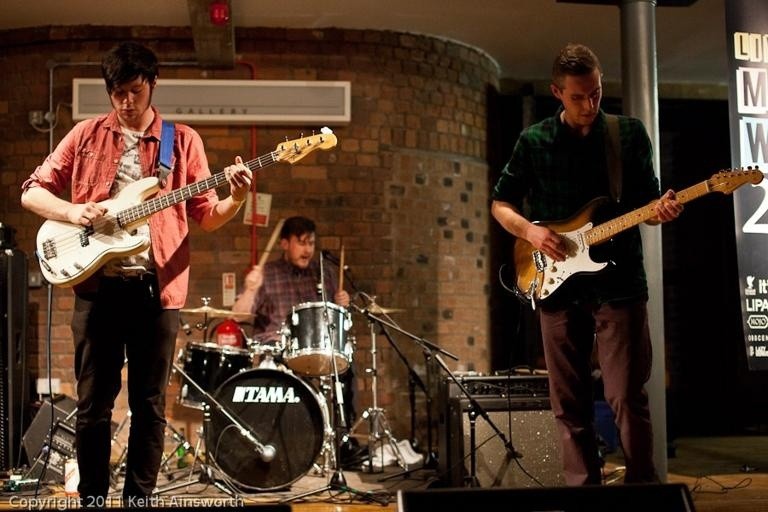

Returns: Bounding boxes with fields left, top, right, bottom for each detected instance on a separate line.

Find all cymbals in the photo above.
left=179, top=306, right=256, bottom=317
left=361, top=303, right=406, bottom=316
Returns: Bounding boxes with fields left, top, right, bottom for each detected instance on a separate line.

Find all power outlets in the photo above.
left=28, top=110, right=43, bottom=124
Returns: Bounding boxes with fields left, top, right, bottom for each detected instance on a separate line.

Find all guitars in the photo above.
left=36, top=128, right=337, bottom=288
left=512, top=166, right=764, bottom=306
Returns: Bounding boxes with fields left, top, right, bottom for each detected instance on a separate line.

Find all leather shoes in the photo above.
left=338, top=441, right=369, bottom=464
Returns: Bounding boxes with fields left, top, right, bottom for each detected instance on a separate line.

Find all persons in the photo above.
left=489, top=42, right=685, bottom=488
left=230, top=214, right=376, bottom=470
left=19, top=39, right=255, bottom=510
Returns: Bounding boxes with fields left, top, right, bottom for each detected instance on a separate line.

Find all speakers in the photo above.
left=397, top=484, right=696, bottom=512
left=440, top=376, right=595, bottom=488
left=23, top=394, right=119, bottom=484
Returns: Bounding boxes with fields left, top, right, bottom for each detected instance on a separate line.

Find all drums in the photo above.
left=181, top=342, right=252, bottom=412
left=202, top=368, right=328, bottom=492
left=287, top=304, right=352, bottom=379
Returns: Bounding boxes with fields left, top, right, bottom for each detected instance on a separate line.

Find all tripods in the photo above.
left=339, top=321, right=409, bottom=476
left=278, top=373, right=388, bottom=505
left=111, top=411, right=203, bottom=486
left=152, top=425, right=237, bottom=501
left=379, top=347, right=439, bottom=481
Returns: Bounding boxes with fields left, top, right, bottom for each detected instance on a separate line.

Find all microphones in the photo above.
left=321, top=251, right=351, bottom=271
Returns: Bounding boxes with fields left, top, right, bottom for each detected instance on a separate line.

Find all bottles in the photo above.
left=177, top=427, right=189, bottom=467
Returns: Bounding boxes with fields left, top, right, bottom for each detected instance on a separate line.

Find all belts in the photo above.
left=103, top=265, right=156, bottom=279
